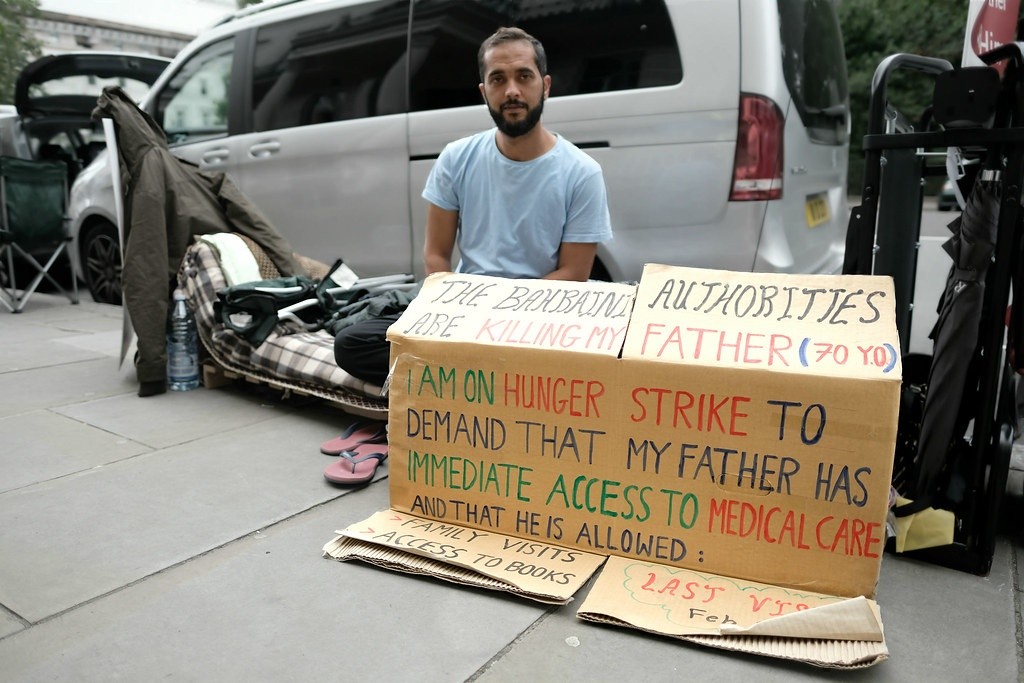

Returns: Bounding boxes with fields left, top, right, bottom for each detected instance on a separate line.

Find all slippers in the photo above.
left=320, top=421, right=387, bottom=455
left=324, top=443, right=388, bottom=485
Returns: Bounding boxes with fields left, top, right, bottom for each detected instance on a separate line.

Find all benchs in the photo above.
left=178, top=231, right=419, bottom=421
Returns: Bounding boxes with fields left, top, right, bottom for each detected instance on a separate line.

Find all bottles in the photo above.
left=165, top=294, right=200, bottom=391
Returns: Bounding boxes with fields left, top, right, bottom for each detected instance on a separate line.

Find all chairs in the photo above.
left=0, top=154, right=81, bottom=316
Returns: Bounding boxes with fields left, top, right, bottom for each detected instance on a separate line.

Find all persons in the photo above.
left=334, top=27, right=613, bottom=390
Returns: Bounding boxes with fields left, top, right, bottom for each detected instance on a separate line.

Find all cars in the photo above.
left=63, top=0, right=853, bottom=306
